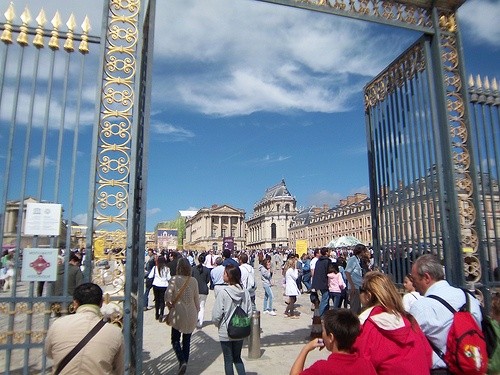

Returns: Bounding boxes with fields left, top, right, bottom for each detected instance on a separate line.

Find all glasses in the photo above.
left=359, top=286, right=366, bottom=293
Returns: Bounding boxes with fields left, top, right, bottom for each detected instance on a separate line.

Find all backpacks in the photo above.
left=244, top=265, right=256, bottom=292
left=223, top=299, right=251, bottom=338
left=426, top=288, right=489, bottom=375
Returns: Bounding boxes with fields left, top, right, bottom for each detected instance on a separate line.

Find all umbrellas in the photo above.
left=327, top=236, right=363, bottom=248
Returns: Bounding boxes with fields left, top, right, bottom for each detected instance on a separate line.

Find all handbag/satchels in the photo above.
left=147, top=267, right=156, bottom=287
left=162, top=307, right=171, bottom=325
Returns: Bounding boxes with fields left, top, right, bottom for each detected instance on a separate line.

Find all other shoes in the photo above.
left=178, top=362, right=186, bottom=375
left=159, top=315, right=162, bottom=322
left=156, top=314, right=159, bottom=319
left=268, top=311, right=276, bottom=316
left=264, top=310, right=268, bottom=314
left=290, top=315, right=300, bottom=319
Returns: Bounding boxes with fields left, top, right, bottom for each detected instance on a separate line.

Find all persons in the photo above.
left=36, top=244, right=125, bottom=319
left=402, top=255, right=500, bottom=375
left=212, top=264, right=252, bottom=375
left=289, top=308, right=377, bottom=375
left=0, top=249, right=22, bottom=291
left=44, top=282, right=125, bottom=375
left=145, top=243, right=437, bottom=322
left=352, top=271, right=432, bottom=375
left=164, top=258, right=201, bottom=375
